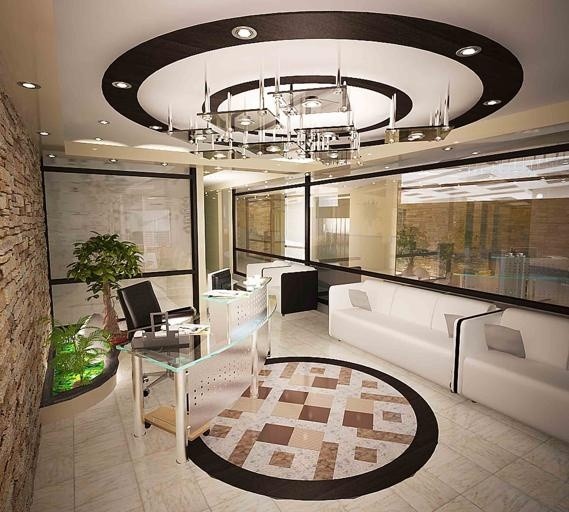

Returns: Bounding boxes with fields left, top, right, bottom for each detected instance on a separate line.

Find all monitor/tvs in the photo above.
left=208, top=267, right=234, bottom=290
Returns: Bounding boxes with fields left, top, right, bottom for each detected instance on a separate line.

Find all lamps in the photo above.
left=167, top=46, right=456, bottom=161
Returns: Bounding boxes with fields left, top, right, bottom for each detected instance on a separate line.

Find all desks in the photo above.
left=116, top=295, right=277, bottom=464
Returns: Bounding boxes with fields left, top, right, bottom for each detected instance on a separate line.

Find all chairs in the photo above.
left=246, top=259, right=318, bottom=316
left=117, top=281, right=197, bottom=394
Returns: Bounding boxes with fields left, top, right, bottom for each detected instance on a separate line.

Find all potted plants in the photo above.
left=66, top=231, right=144, bottom=346
